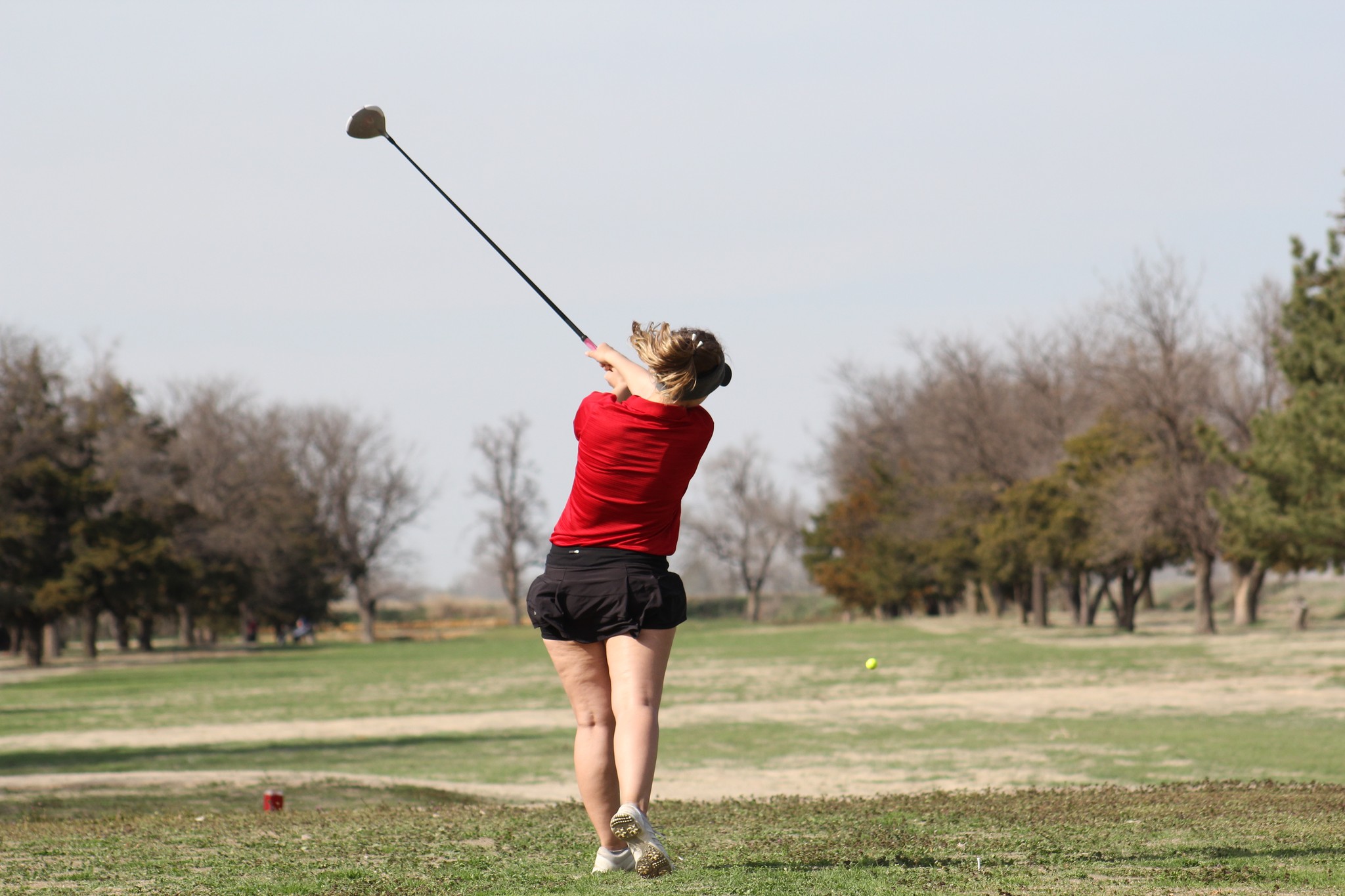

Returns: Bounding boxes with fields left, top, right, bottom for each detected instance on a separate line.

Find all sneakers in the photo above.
left=591, top=846, right=636, bottom=875
left=610, top=803, right=673, bottom=879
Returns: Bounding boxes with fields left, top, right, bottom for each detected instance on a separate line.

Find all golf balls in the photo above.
left=866, top=656, right=877, bottom=671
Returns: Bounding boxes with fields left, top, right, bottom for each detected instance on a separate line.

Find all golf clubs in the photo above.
left=343, top=107, right=619, bottom=373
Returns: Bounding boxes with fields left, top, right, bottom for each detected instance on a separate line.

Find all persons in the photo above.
left=294, top=620, right=311, bottom=646
left=524, top=322, right=730, bottom=879
left=241, top=611, right=259, bottom=648
